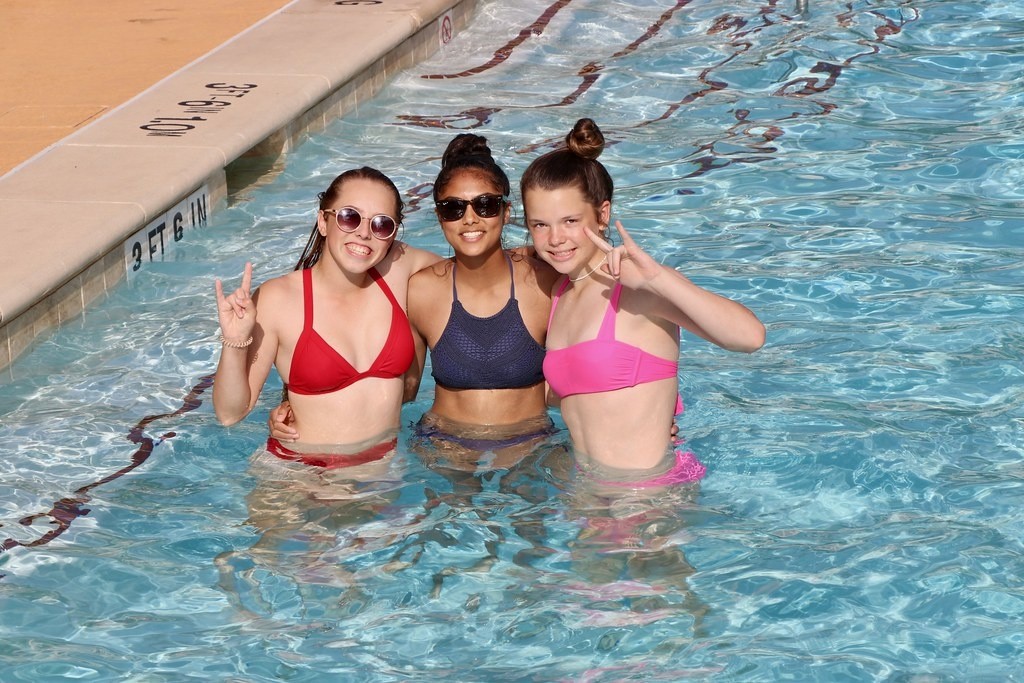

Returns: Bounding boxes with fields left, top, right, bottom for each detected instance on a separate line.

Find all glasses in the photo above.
left=436, top=193, right=508, bottom=221
left=324, top=207, right=399, bottom=240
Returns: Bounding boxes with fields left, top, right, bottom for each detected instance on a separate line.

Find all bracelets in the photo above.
left=220, top=335, right=253, bottom=348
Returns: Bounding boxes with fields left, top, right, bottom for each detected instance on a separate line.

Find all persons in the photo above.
left=504, top=118, right=766, bottom=469
left=213, top=165, right=445, bottom=445
left=266, top=132, right=682, bottom=445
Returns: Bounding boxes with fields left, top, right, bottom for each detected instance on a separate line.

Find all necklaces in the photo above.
left=568, top=256, right=606, bottom=282
